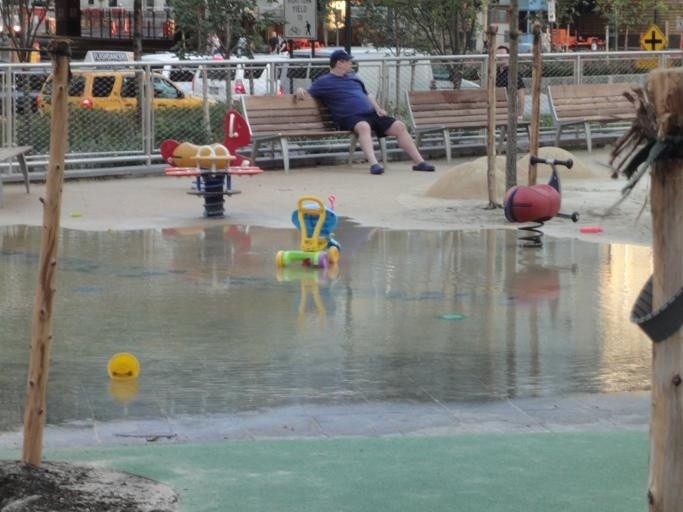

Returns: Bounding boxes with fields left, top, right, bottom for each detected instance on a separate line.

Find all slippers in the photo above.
left=412, top=160, right=436, bottom=171
left=370, top=163, right=385, bottom=174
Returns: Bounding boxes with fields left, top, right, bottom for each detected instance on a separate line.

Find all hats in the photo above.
left=330, top=50, right=355, bottom=62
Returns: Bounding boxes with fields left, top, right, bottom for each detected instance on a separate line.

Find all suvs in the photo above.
left=36, top=50, right=221, bottom=115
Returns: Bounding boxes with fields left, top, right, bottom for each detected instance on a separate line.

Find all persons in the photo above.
left=294, top=49, right=435, bottom=174
left=493, top=45, right=525, bottom=117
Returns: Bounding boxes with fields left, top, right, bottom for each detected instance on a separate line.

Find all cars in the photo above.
left=498, top=33, right=551, bottom=54
left=138, top=46, right=482, bottom=118
left=0, top=71, right=49, bottom=118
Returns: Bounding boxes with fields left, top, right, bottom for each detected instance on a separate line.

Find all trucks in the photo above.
left=550, top=28, right=606, bottom=51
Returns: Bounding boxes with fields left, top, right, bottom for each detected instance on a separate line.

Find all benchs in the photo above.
left=547, top=84, right=648, bottom=154
left=0, top=145, right=35, bottom=193
left=240, top=95, right=387, bottom=175
left=405, top=87, right=543, bottom=164
